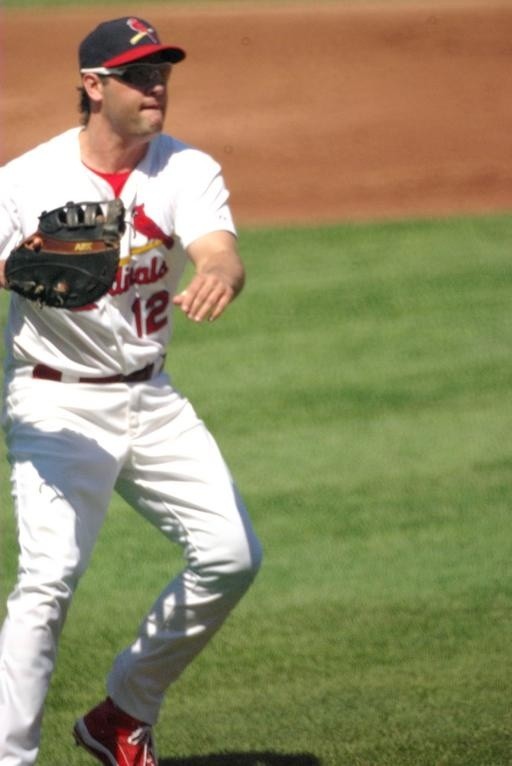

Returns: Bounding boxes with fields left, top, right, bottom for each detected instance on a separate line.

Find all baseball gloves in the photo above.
left=4, top=200, right=124, bottom=307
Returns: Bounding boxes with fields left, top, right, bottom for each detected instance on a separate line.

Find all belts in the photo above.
left=30, top=354, right=170, bottom=386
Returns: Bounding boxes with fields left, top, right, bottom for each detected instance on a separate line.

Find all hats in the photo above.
left=79, top=16, right=186, bottom=68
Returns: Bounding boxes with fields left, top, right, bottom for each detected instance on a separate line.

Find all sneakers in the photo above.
left=71, top=695, right=161, bottom=766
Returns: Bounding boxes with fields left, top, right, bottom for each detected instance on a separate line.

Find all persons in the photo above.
left=0, top=16, right=269, bottom=765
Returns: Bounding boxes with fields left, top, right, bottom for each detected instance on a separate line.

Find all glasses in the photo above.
left=80, top=56, right=173, bottom=87
